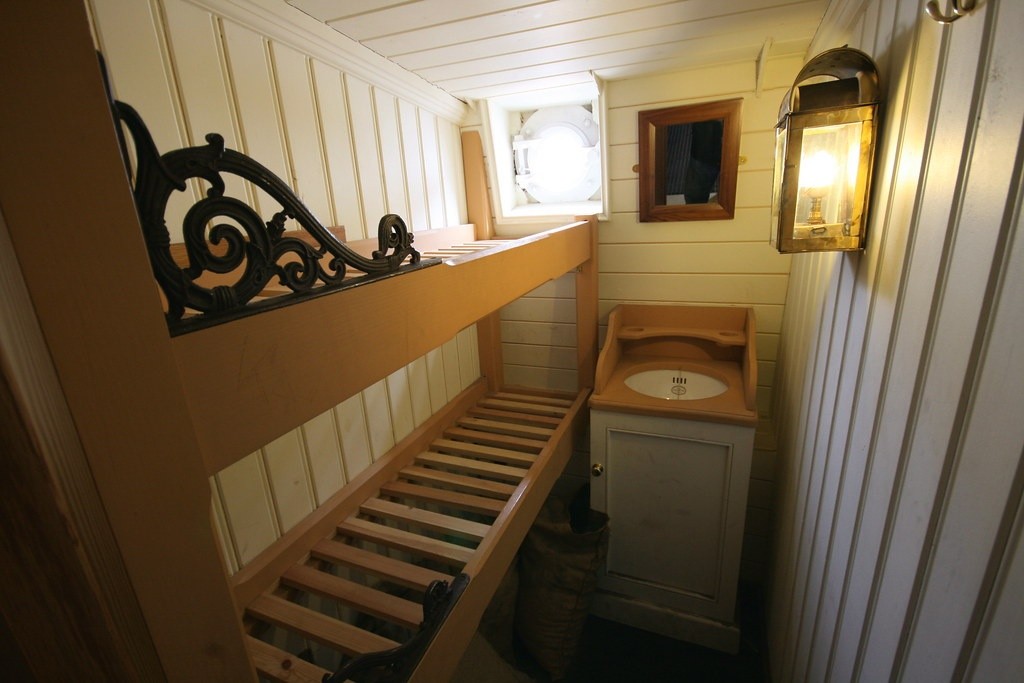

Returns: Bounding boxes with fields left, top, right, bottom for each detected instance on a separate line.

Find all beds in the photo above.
left=0, top=0, right=602, bottom=683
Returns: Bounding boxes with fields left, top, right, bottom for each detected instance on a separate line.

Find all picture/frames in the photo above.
left=638, top=98, right=743, bottom=223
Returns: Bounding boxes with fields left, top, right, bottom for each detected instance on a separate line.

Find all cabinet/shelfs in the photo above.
left=589, top=409, right=756, bottom=655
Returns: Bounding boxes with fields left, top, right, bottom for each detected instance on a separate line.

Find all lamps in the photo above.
left=768, top=103, right=881, bottom=255
